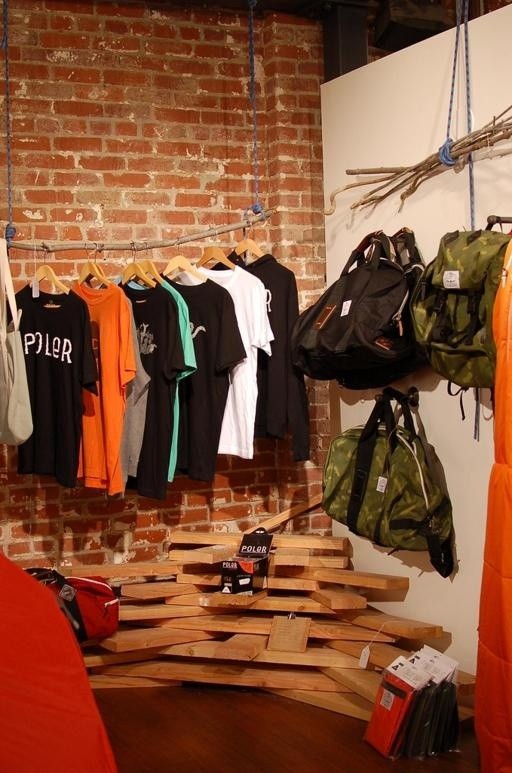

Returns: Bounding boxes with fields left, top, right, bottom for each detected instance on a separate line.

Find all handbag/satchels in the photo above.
left=289, top=222, right=430, bottom=389
left=0, top=238, right=36, bottom=447
left=319, top=387, right=455, bottom=577
left=23, top=567, right=120, bottom=643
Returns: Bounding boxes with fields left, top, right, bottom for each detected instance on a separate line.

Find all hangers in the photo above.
left=28, top=219, right=265, bottom=309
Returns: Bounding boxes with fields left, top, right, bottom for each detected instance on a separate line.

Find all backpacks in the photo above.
left=411, top=220, right=509, bottom=391
